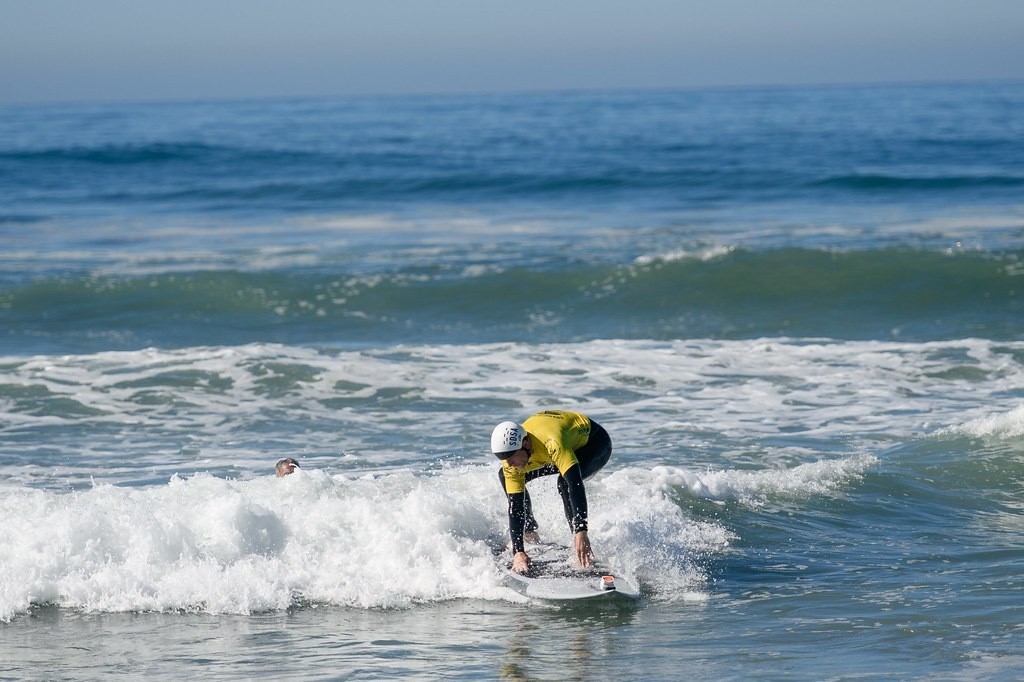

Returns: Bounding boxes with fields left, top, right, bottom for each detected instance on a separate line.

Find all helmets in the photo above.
left=490, top=421, right=527, bottom=453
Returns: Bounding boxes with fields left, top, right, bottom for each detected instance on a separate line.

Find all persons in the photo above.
left=276, top=457, right=299, bottom=478
left=491, top=411, right=613, bottom=572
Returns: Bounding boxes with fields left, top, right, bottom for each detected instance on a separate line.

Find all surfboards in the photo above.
left=488, top=540, right=640, bottom=604
